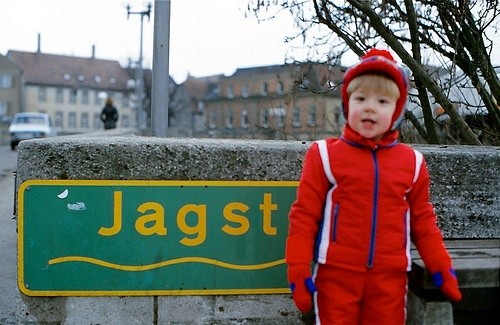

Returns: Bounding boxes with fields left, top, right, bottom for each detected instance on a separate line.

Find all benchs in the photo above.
left=407, top=238, right=500, bottom=325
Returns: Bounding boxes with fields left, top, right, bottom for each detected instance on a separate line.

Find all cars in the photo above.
left=8, top=111, right=59, bottom=151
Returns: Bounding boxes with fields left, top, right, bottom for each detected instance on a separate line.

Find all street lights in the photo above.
left=125, top=2, right=153, bottom=130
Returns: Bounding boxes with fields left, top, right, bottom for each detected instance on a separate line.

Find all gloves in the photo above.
left=286, top=265, right=317, bottom=313
left=429, top=262, right=462, bottom=302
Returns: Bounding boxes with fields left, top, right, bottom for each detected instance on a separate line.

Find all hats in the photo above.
left=339, top=47, right=408, bottom=134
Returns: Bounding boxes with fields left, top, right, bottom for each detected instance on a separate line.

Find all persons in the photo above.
left=101, top=98, right=119, bottom=130
left=286, top=49, right=462, bottom=325
